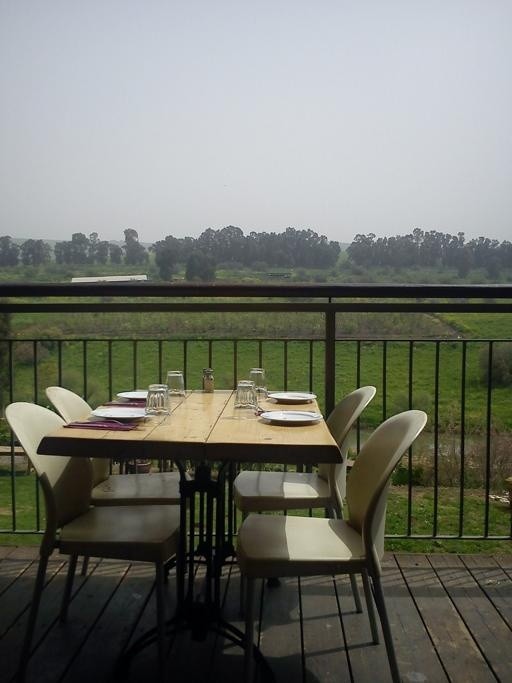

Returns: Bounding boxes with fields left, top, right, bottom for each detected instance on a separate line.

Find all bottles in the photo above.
left=203, top=369, right=215, bottom=393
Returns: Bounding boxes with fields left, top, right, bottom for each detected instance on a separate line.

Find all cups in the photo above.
left=143, top=383, right=170, bottom=427
left=248, top=367, right=268, bottom=402
left=232, top=379, right=259, bottom=420
left=166, top=370, right=186, bottom=406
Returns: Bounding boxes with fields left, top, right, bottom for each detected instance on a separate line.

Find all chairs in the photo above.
left=47, top=385, right=190, bottom=605
left=6, top=402, right=180, bottom=663
left=224, top=385, right=378, bottom=613
left=235, top=409, right=428, bottom=683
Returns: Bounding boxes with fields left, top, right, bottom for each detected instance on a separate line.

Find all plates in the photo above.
left=91, top=406, right=155, bottom=420
left=260, top=409, right=323, bottom=423
left=116, top=390, right=164, bottom=400
left=267, top=391, right=318, bottom=403
